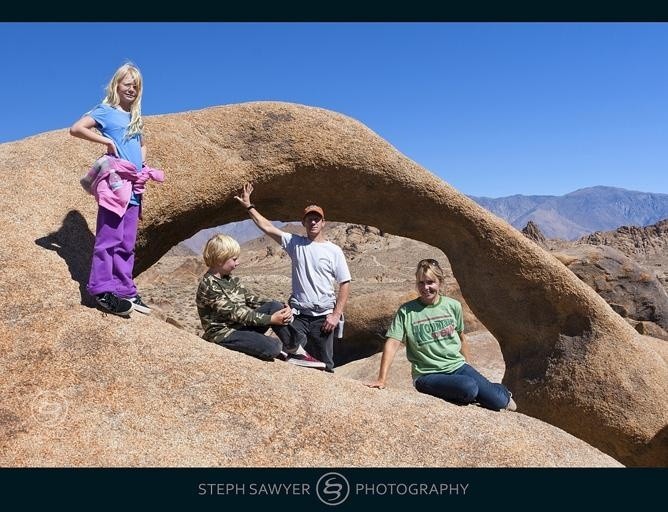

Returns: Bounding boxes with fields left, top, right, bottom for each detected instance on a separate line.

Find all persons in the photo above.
left=68, top=58, right=165, bottom=315
left=364, top=258, right=517, bottom=411
left=194, top=232, right=328, bottom=370
left=233, top=181, right=354, bottom=374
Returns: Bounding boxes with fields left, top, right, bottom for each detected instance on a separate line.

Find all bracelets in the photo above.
left=244, top=204, right=256, bottom=210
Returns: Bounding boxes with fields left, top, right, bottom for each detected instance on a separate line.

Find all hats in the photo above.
left=302, top=204, right=324, bottom=220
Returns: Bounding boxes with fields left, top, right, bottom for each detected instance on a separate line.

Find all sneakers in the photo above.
left=91, top=291, right=134, bottom=315
left=508, top=394, right=517, bottom=411
left=129, top=295, right=151, bottom=316
left=278, top=347, right=327, bottom=370
